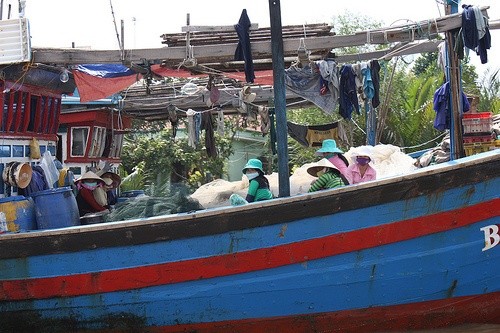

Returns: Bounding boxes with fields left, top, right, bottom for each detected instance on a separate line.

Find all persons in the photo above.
left=308, top=139, right=377, bottom=193
left=230, top=159, right=274, bottom=206
left=73, top=171, right=121, bottom=216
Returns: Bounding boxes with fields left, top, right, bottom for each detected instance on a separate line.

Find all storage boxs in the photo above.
left=462, top=112, right=496, bottom=157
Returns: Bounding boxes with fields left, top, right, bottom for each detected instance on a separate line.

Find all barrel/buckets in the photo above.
left=118, top=189, right=145, bottom=202
left=29, top=186, right=81, bottom=229
left=0, top=195, right=34, bottom=235
left=79, top=215, right=105, bottom=225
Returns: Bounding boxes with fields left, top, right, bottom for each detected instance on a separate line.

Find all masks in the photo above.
left=103, top=178, right=113, bottom=185
left=356, top=158, right=369, bottom=166
left=246, top=172, right=259, bottom=180
left=84, top=182, right=97, bottom=190
left=317, top=168, right=328, bottom=177
left=324, top=152, right=335, bottom=159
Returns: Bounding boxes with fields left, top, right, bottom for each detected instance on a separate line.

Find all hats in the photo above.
left=351, top=148, right=375, bottom=164
left=317, top=140, right=344, bottom=153
left=307, top=157, right=341, bottom=177
left=241, top=159, right=265, bottom=176
left=100, top=172, right=121, bottom=188
left=74, top=171, right=105, bottom=187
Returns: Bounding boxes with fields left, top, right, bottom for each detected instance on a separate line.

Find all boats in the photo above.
left=0, top=0, right=500, bottom=333
